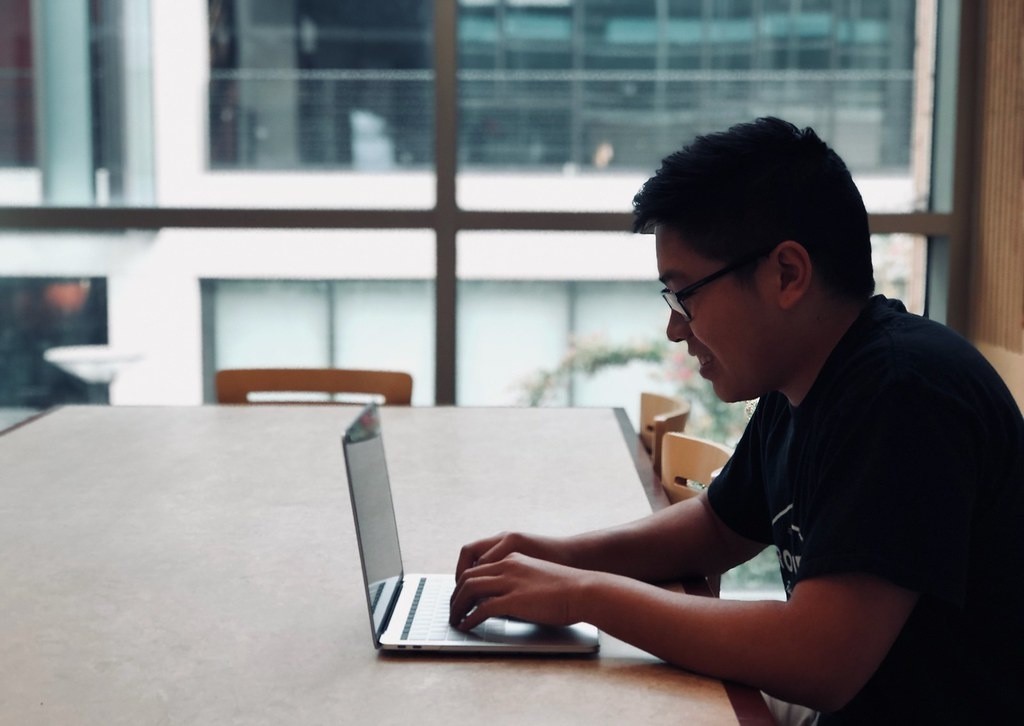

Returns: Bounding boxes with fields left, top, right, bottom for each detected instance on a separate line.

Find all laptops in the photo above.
left=341, top=402, right=600, bottom=653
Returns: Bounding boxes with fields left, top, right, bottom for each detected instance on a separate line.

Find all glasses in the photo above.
left=662, top=248, right=771, bottom=321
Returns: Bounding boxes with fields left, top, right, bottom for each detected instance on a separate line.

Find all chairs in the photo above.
left=640, top=391, right=735, bottom=504
left=216, top=369, right=412, bottom=405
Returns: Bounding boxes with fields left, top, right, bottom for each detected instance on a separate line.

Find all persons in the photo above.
left=450, top=115, right=1024, bottom=725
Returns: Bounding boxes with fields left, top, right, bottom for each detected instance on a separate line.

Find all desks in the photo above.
left=0, top=400, right=772, bottom=726
